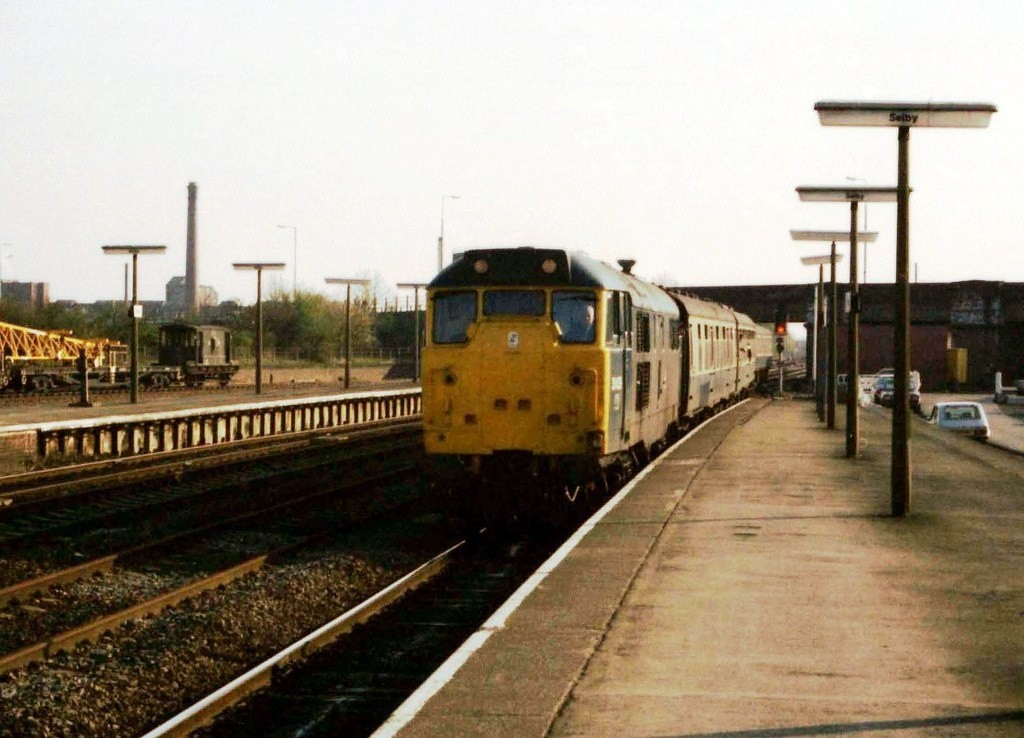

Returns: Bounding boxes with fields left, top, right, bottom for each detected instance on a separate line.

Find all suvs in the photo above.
left=871, top=367, right=920, bottom=413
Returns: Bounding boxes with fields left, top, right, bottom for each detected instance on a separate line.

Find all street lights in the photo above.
left=395, top=283, right=429, bottom=385
left=102, top=244, right=169, bottom=405
left=813, top=97, right=999, bottom=521
left=796, top=182, right=914, bottom=462
left=438, top=194, right=462, bottom=272
left=326, top=276, right=372, bottom=389
left=801, top=253, right=845, bottom=420
left=788, top=228, right=878, bottom=431
left=279, top=224, right=296, bottom=298
left=233, top=263, right=286, bottom=395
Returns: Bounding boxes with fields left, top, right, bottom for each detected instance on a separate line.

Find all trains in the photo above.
left=420, top=248, right=796, bottom=512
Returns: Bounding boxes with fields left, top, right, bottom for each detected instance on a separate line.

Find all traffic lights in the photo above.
left=772, top=316, right=787, bottom=337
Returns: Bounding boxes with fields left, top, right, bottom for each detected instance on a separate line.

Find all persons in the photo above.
left=560, top=305, right=596, bottom=343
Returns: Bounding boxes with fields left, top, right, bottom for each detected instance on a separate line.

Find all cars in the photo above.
left=927, top=402, right=990, bottom=445
